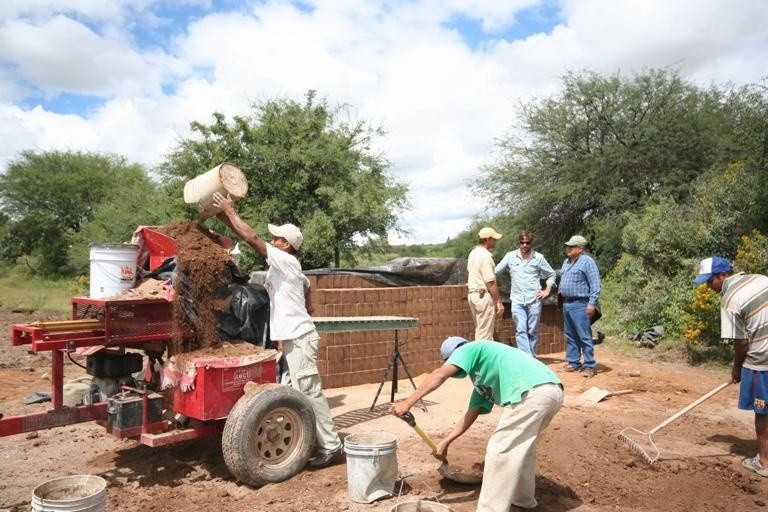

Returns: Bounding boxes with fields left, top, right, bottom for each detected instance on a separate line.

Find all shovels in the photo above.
left=579, top=386, right=633, bottom=404
left=400, top=411, right=483, bottom=484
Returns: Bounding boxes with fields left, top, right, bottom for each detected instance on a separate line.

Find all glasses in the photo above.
left=519, top=241, right=531, bottom=245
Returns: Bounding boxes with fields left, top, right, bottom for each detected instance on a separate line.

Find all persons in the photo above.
left=693, top=256, right=768, bottom=479
left=209, top=192, right=344, bottom=471
left=465, top=226, right=507, bottom=343
left=492, top=231, right=557, bottom=358
left=555, top=236, right=602, bottom=381
left=393, top=332, right=568, bottom=511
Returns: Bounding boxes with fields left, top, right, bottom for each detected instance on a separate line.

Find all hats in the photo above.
left=564, top=235, right=587, bottom=248
left=691, top=256, right=734, bottom=286
left=265, top=221, right=304, bottom=252
left=439, top=335, right=469, bottom=360
left=478, top=226, right=503, bottom=241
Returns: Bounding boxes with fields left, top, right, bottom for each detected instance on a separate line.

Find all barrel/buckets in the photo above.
left=89, top=242, right=140, bottom=299
left=31, top=474, right=108, bottom=512
left=343, top=431, right=397, bottom=504
left=389, top=473, right=455, bottom=512
left=184, top=162, right=249, bottom=224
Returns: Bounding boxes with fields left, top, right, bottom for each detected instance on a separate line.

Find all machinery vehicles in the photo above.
left=0, top=222, right=316, bottom=489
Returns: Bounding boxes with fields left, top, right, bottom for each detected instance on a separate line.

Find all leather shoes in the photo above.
left=308, top=445, right=344, bottom=469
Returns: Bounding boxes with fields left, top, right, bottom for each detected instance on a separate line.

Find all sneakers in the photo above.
left=742, top=452, right=768, bottom=477
left=555, top=363, right=581, bottom=372
left=581, top=367, right=596, bottom=379
